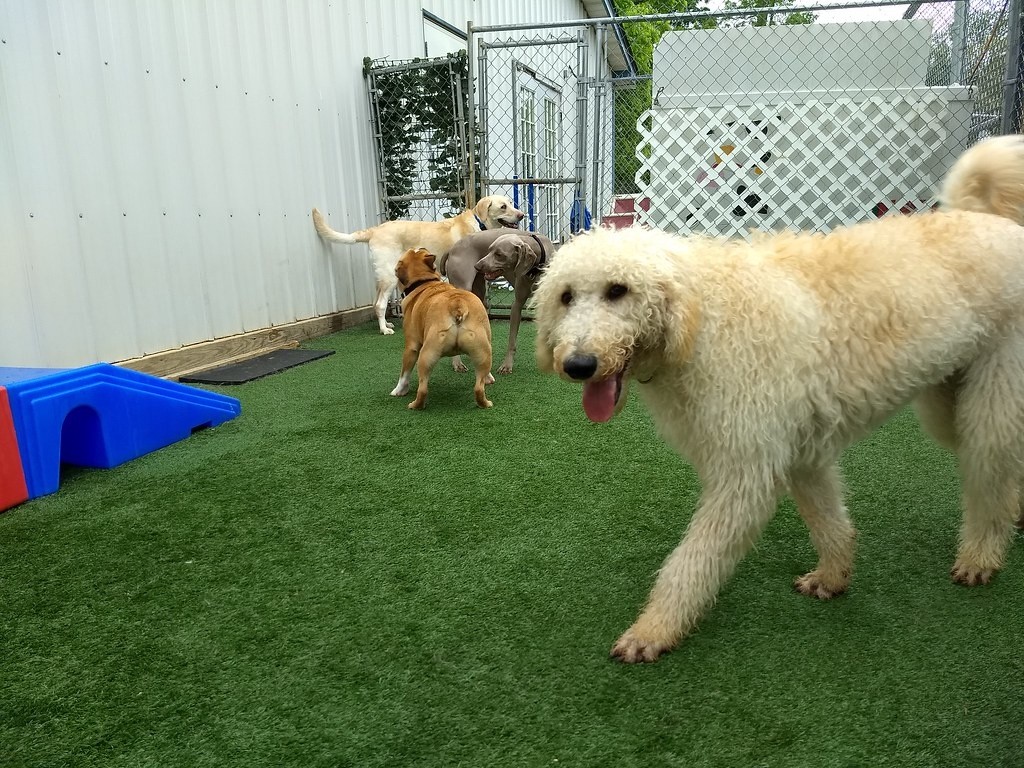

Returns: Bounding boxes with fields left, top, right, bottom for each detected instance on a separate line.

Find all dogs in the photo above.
left=439, top=228, right=555, bottom=375
left=388, top=247, right=496, bottom=411
left=311, top=193, right=525, bottom=336
left=532, top=133, right=1024, bottom=666
